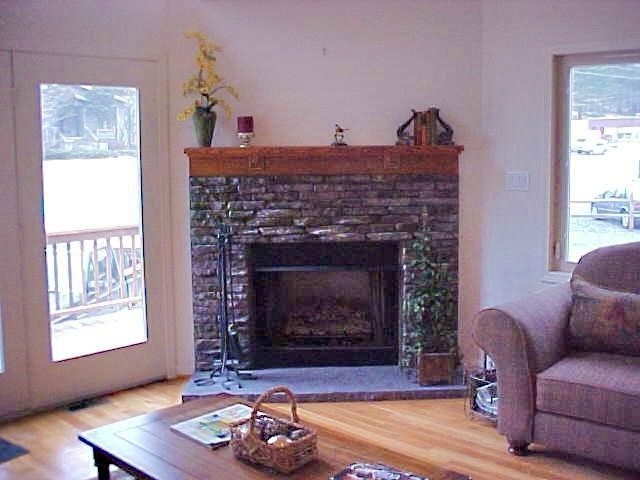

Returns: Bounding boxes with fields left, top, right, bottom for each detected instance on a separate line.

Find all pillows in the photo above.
left=569, top=273, right=640, bottom=354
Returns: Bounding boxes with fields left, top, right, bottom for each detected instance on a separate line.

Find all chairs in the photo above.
left=471, top=239, right=640, bottom=469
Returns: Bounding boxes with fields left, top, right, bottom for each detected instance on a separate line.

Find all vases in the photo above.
left=191, top=108, right=218, bottom=147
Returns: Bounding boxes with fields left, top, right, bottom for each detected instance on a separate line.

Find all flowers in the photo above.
left=164, top=28, right=245, bottom=120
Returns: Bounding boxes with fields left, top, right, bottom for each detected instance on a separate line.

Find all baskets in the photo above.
left=228, top=386, right=319, bottom=475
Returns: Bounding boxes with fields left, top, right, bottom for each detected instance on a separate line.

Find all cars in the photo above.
left=590, top=187, right=640, bottom=228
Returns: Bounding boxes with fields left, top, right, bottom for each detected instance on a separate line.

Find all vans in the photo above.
left=574, top=129, right=601, bottom=155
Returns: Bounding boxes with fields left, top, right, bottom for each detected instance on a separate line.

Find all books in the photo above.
left=170, top=402, right=267, bottom=451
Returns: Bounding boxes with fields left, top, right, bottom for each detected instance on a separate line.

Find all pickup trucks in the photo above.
left=50, top=245, right=143, bottom=323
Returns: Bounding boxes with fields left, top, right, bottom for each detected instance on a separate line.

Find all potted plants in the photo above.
left=396, top=225, right=463, bottom=385
